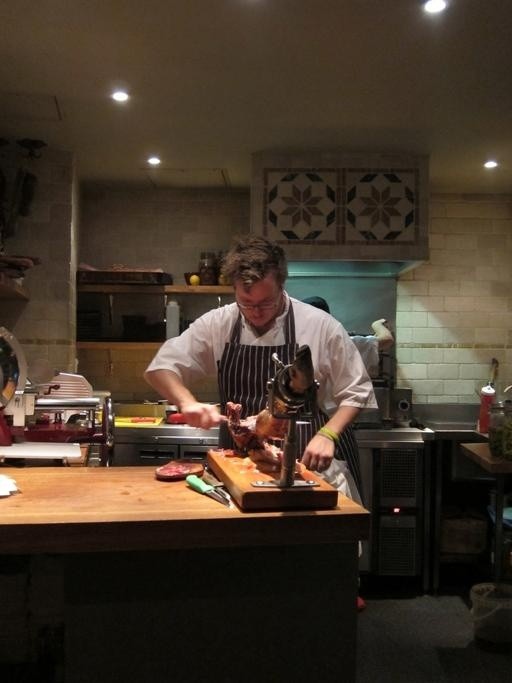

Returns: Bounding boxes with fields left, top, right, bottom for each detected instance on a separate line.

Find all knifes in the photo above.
left=116, top=418, right=156, bottom=424
left=184, top=469, right=232, bottom=509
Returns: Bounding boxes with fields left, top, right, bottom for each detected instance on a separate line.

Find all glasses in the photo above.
left=237, top=302, right=279, bottom=310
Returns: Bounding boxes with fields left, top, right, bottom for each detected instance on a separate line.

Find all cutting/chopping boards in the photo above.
left=115, top=416, right=163, bottom=428
left=206, top=441, right=340, bottom=513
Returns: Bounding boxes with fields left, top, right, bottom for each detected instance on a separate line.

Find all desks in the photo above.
left=0, top=462, right=372, bottom=682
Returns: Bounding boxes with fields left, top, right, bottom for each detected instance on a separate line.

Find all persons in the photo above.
left=143, top=232, right=379, bottom=557
left=302, top=295, right=396, bottom=611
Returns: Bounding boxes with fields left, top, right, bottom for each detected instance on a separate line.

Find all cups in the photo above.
left=183, top=271, right=199, bottom=286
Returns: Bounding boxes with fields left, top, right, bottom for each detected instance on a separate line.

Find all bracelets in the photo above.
left=318, top=426, right=341, bottom=446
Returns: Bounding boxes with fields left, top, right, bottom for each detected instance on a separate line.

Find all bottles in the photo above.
left=486, top=399, right=512, bottom=463
left=199, top=250, right=218, bottom=285
left=479, top=384, right=497, bottom=434
left=166, top=299, right=180, bottom=341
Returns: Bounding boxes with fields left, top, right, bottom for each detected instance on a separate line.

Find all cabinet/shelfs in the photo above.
left=76, top=283, right=236, bottom=351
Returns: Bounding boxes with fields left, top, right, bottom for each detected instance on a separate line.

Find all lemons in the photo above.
left=190, top=275, right=200, bottom=285
